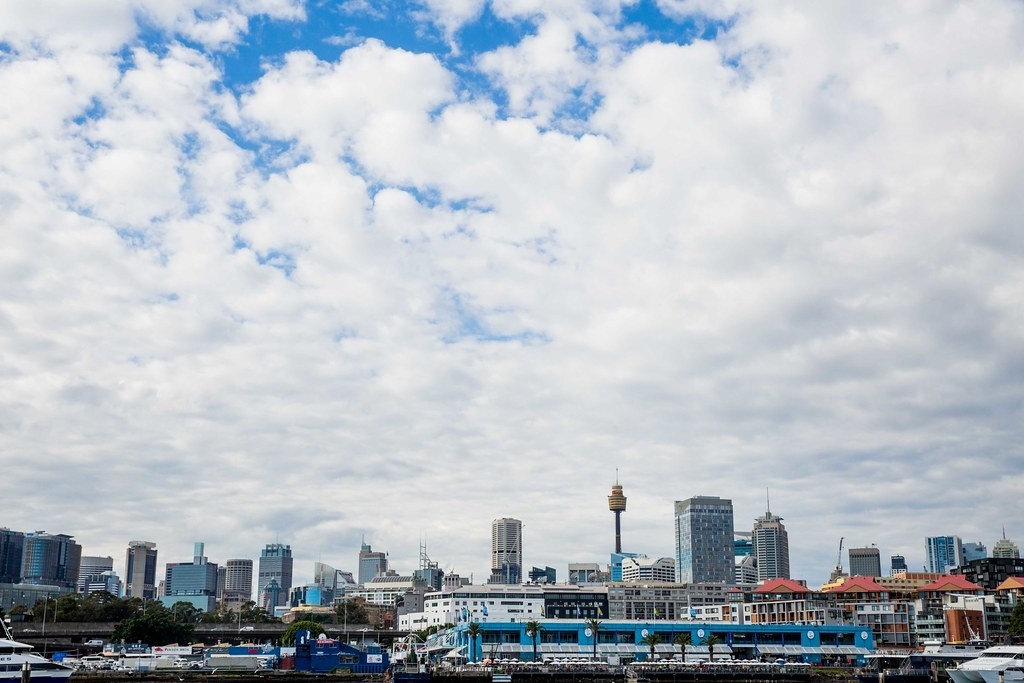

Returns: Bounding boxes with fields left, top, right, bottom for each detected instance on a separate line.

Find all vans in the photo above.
left=240, top=626, right=254, bottom=631
left=83, top=639, right=103, bottom=646
left=80, top=655, right=105, bottom=668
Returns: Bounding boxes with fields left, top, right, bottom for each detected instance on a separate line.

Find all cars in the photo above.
left=104, top=658, right=120, bottom=670
left=174, top=657, right=204, bottom=670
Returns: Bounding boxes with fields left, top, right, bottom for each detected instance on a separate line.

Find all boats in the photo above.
left=0, top=617, right=77, bottom=683
left=864, top=635, right=994, bottom=675
left=943, top=645, right=1024, bottom=683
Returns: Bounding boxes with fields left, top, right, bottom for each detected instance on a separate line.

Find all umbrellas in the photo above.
left=629, top=658, right=811, bottom=666
left=443, top=650, right=609, bottom=667
